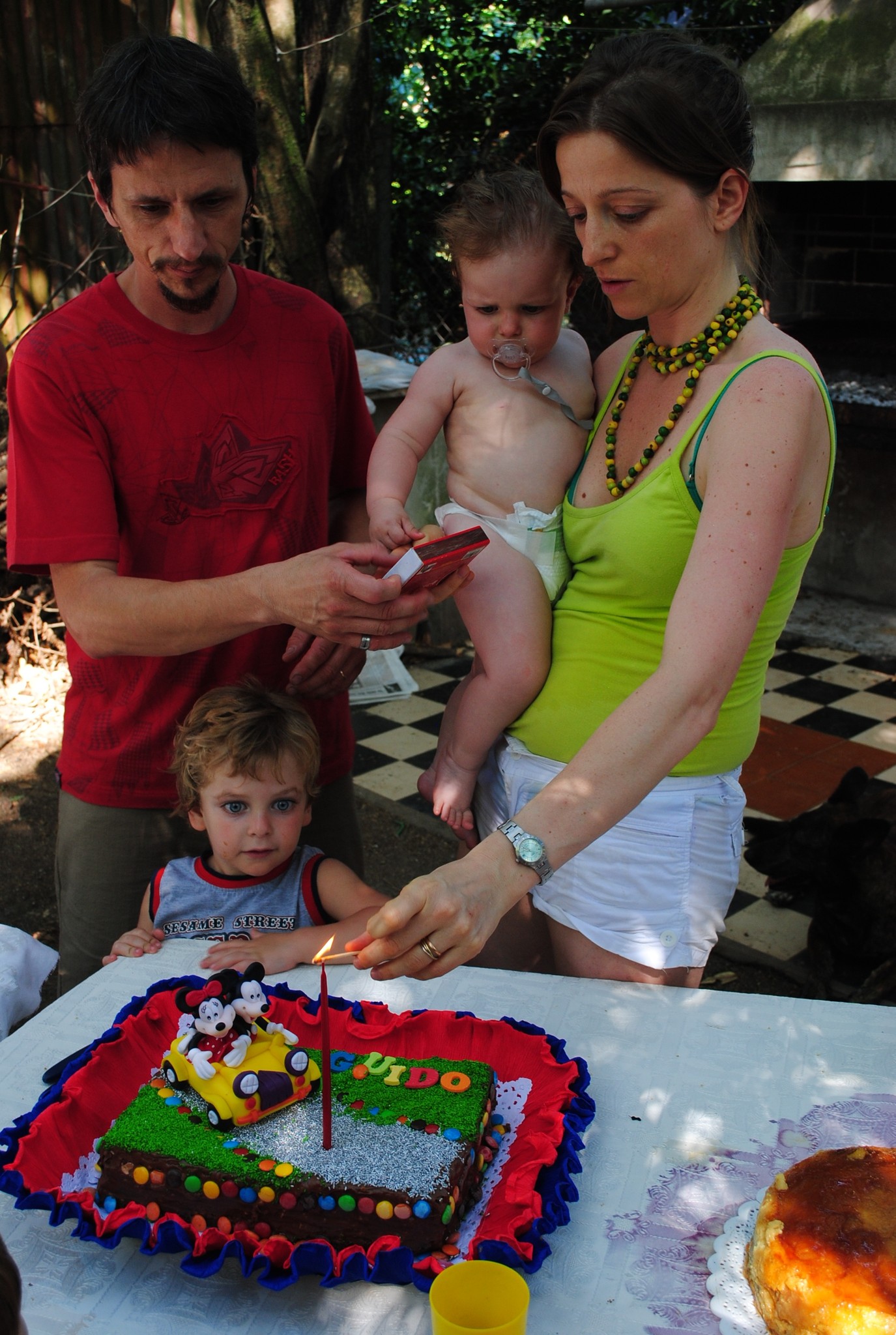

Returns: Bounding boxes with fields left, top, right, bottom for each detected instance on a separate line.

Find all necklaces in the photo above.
left=588, top=271, right=764, bottom=499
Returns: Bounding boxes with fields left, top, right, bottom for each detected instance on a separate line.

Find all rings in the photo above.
left=420, top=938, right=442, bottom=961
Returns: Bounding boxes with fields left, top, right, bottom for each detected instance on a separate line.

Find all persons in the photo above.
left=337, top=49, right=845, bottom=984
left=8, top=34, right=382, bottom=986
left=86, top=662, right=394, bottom=982
left=361, top=164, right=600, bottom=832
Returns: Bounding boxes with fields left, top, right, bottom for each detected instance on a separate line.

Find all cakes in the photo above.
left=85, top=959, right=501, bottom=1252
left=741, top=1143, right=895, bottom=1335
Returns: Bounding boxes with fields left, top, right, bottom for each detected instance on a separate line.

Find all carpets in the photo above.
left=344, top=618, right=895, bottom=999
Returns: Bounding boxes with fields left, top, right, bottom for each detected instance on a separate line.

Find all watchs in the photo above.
left=496, top=820, right=559, bottom=889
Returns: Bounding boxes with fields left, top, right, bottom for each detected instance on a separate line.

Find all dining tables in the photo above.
left=0, top=930, right=896, bottom=1335
